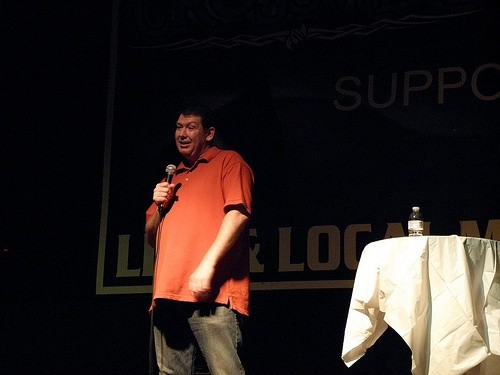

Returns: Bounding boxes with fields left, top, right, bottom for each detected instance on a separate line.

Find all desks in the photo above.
left=365, top=236, right=500, bottom=375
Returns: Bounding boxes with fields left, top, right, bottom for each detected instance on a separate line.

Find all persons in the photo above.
left=145, top=104, right=255, bottom=375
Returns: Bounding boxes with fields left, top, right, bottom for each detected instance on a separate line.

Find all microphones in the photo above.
left=159, top=165, right=176, bottom=212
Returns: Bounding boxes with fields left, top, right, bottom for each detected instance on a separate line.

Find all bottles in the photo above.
left=409, top=207, right=423, bottom=237
